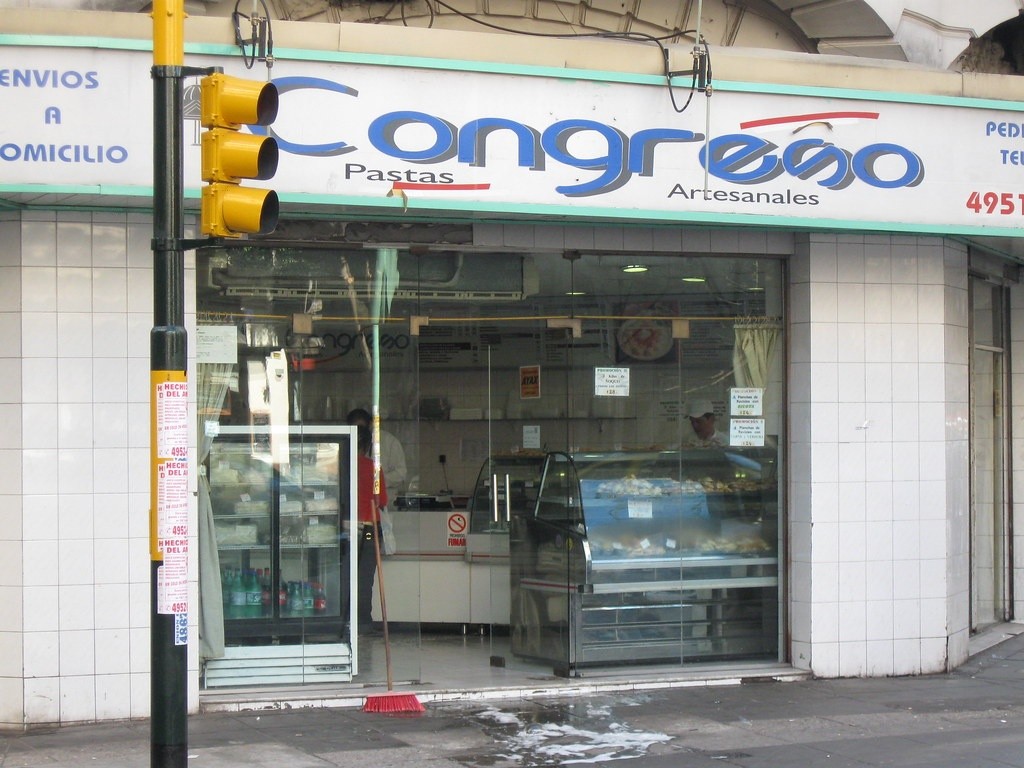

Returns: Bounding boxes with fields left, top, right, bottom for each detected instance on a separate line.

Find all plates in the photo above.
left=618, top=311, right=675, bottom=362
left=485, top=409, right=505, bottom=419
left=449, top=408, right=482, bottom=419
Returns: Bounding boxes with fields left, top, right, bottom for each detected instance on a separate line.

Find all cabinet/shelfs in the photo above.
left=210, top=482, right=340, bottom=552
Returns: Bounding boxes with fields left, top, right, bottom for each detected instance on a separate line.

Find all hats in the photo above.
left=683, top=399, right=714, bottom=418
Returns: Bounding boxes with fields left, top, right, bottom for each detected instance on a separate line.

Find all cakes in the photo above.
left=210, top=464, right=339, bottom=545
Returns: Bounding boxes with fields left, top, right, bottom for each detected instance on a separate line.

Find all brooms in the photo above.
left=363, top=499, right=424, bottom=714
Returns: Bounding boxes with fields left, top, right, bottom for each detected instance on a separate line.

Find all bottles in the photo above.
left=220, top=568, right=272, bottom=619
left=279, top=569, right=288, bottom=617
left=287, top=581, right=327, bottom=615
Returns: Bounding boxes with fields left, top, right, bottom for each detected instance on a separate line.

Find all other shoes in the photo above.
left=358, top=624, right=384, bottom=636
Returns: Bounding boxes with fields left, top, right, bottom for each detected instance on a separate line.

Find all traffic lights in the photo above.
left=200, top=71, right=280, bottom=241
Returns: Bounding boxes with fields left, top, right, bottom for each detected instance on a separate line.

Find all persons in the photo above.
left=345, top=408, right=408, bottom=511
left=683, top=398, right=739, bottom=449
left=340, top=425, right=383, bottom=638
